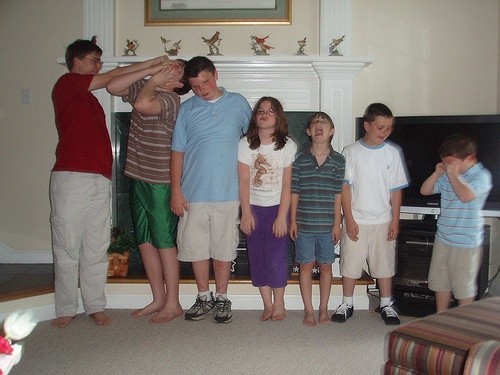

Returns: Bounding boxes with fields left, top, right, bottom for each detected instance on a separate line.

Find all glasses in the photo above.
left=84, top=57, right=104, bottom=66
left=257, top=109, right=275, bottom=116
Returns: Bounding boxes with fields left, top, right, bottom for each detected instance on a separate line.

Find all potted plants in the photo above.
left=107, top=226, right=138, bottom=277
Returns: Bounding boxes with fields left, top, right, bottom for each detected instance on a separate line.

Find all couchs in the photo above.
left=379, top=295, right=500, bottom=375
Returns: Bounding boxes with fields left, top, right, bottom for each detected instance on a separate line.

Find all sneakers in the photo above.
left=212, top=295, right=233, bottom=324
left=184, top=292, right=217, bottom=321
left=378, top=301, right=401, bottom=326
left=330, top=303, right=355, bottom=323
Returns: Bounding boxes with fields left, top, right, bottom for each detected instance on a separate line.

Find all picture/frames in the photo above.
left=144, top=0, right=292, bottom=27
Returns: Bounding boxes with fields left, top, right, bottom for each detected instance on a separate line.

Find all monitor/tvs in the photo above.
left=355, top=115, right=500, bottom=212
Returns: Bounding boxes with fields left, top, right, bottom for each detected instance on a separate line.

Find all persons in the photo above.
left=47, top=38, right=169, bottom=328
left=171, top=56, right=255, bottom=323
left=237, top=94, right=297, bottom=321
left=291, top=110, right=345, bottom=327
left=106, top=59, right=191, bottom=325
left=331, top=102, right=410, bottom=325
left=420, top=132, right=493, bottom=313
